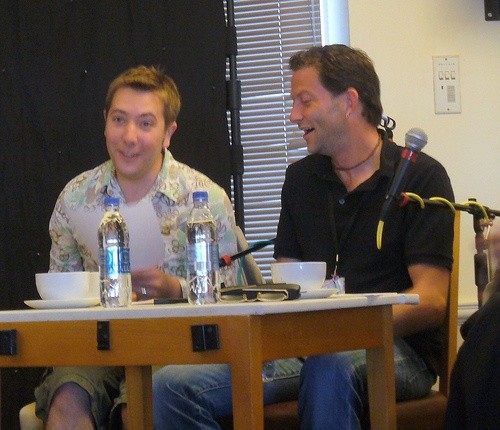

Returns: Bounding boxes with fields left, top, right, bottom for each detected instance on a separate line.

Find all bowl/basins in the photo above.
left=270, top=261, right=327, bottom=289
left=34, top=272, right=99, bottom=299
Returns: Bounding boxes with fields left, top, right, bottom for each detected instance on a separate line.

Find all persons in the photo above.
left=152, top=44, right=455, bottom=430
left=35, top=65, right=236, bottom=430
left=446, top=218, right=500, bottom=430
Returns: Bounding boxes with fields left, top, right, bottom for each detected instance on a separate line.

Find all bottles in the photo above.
left=97, top=197, right=132, bottom=308
left=184, top=191, right=221, bottom=305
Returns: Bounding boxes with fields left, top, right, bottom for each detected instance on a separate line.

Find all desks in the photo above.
left=0, top=291, right=420, bottom=430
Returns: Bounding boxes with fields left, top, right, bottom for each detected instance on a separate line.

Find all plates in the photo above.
left=23, top=298, right=102, bottom=309
left=299, top=288, right=338, bottom=299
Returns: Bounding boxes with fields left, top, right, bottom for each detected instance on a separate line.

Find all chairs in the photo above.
left=19, top=210, right=460, bottom=430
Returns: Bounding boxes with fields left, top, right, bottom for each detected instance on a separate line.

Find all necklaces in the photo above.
left=331, top=133, right=381, bottom=171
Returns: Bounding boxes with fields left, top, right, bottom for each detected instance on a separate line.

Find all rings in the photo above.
left=140, top=287, right=147, bottom=298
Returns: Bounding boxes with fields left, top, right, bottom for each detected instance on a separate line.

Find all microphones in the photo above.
left=378, top=127, right=428, bottom=223
